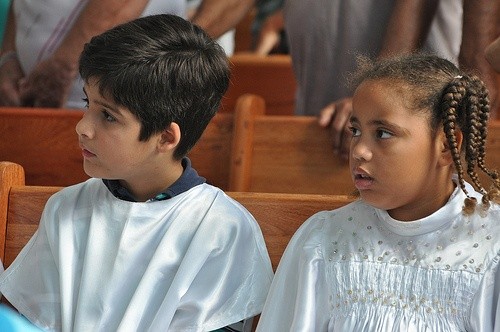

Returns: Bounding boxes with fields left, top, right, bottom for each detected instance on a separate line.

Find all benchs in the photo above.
left=1, top=162, right=358, bottom=331
left=1, top=94, right=499, bottom=201
left=217, top=55, right=295, bottom=114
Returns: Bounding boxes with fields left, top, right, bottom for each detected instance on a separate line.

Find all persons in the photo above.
left=0, top=14, right=275, bottom=332
left=255, top=53, right=500, bottom=332
left=0, top=0, right=500, bottom=163
